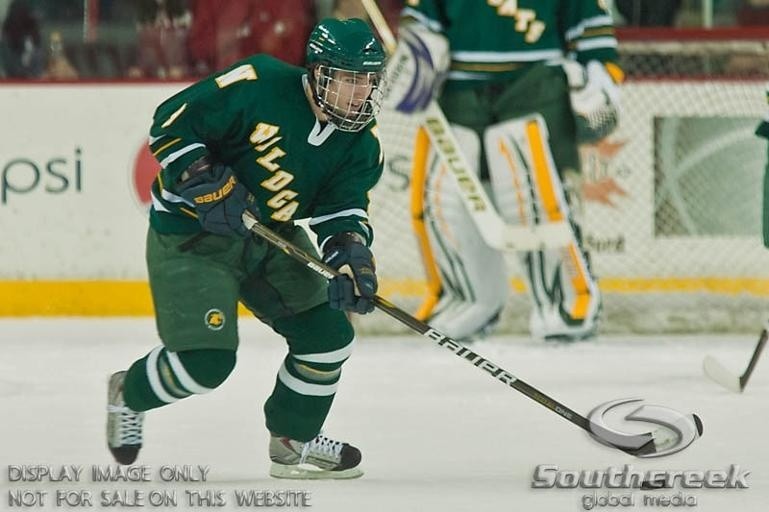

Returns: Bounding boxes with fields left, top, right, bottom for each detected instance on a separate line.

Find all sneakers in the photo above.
left=269, top=433, right=361, bottom=472
left=107, top=371, right=144, bottom=465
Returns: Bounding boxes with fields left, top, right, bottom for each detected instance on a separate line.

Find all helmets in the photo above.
left=305, top=18, right=390, bottom=134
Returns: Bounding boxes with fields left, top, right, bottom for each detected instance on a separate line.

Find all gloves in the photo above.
left=175, top=162, right=264, bottom=239
left=320, top=232, right=377, bottom=314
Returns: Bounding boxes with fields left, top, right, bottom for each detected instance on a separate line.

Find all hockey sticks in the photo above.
left=362, top=0, right=575, bottom=252
left=702, top=312, right=769, bottom=394
left=242, top=209, right=702, bottom=456
